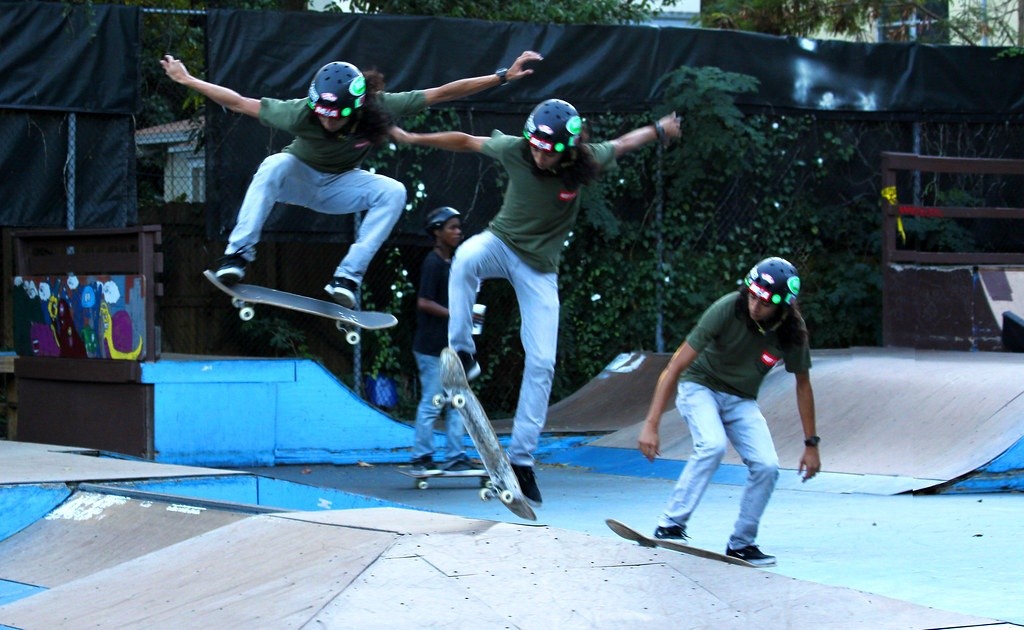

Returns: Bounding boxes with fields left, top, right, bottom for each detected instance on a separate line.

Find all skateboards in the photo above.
left=202, top=268, right=398, bottom=346
left=396, top=466, right=491, bottom=489
left=432, top=347, right=539, bottom=522
left=604, top=516, right=778, bottom=569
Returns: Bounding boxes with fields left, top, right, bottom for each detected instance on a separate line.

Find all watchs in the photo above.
left=496, top=67, right=511, bottom=87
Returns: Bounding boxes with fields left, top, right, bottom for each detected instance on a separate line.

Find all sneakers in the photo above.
left=412, top=455, right=444, bottom=476
left=443, top=453, right=487, bottom=477
left=510, top=462, right=543, bottom=507
left=725, top=542, right=777, bottom=565
left=654, top=524, right=694, bottom=545
left=324, top=277, right=358, bottom=309
left=216, top=245, right=251, bottom=284
left=457, top=349, right=481, bottom=381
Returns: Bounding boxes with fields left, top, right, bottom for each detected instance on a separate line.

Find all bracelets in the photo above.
left=804, top=436, right=820, bottom=447
left=653, top=120, right=666, bottom=140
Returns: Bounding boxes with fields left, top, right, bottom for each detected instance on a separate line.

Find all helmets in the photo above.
left=424, top=206, right=460, bottom=240
left=523, top=99, right=581, bottom=155
left=306, top=61, right=366, bottom=117
left=744, top=257, right=801, bottom=307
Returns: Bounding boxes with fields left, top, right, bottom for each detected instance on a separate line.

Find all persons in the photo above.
left=389, top=99, right=682, bottom=508
left=639, top=257, right=821, bottom=565
left=411, top=207, right=485, bottom=477
left=160, top=51, right=544, bottom=307
